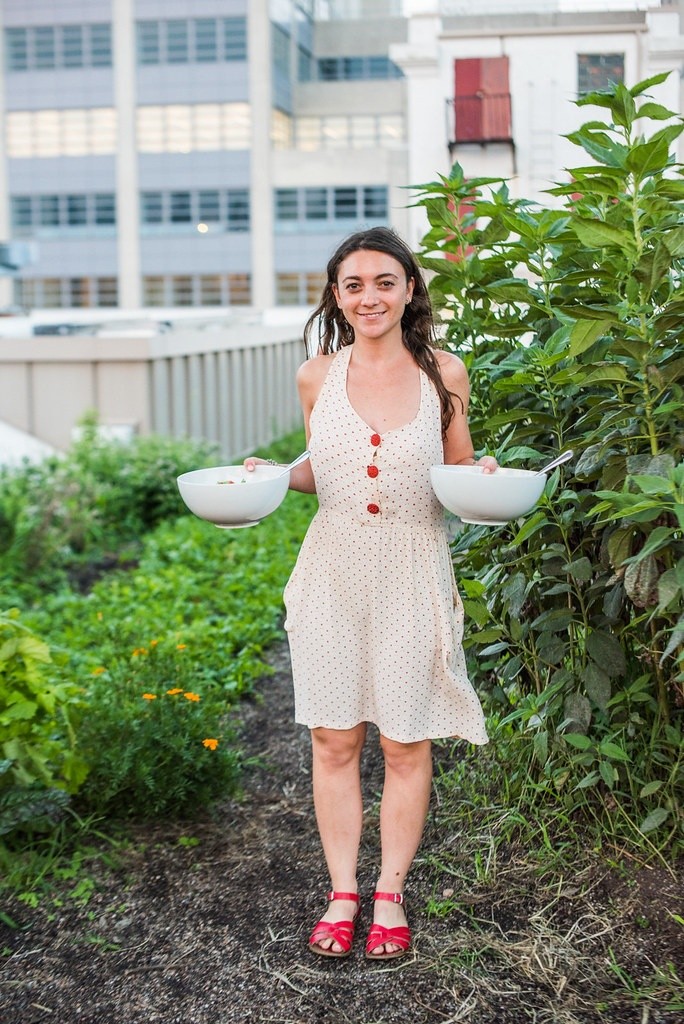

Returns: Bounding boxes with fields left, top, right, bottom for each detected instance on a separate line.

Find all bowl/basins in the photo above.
left=176, top=465, right=291, bottom=529
left=430, top=464, right=547, bottom=525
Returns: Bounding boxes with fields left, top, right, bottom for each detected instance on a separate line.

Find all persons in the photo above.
left=243, top=226, right=497, bottom=961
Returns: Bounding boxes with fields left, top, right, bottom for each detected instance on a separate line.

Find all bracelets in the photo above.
left=267, top=458, right=277, bottom=466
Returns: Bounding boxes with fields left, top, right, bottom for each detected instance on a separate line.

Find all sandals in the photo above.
left=366, top=892, right=412, bottom=959
left=309, top=892, right=362, bottom=958
